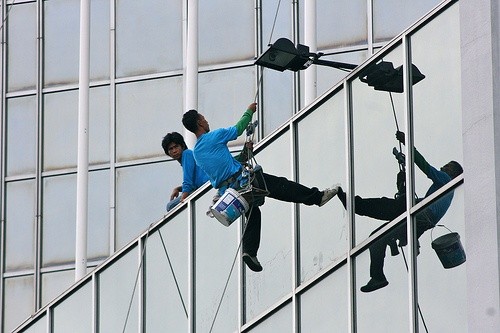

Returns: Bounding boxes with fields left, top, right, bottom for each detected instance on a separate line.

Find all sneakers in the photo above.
left=319, top=187, right=339, bottom=207
left=242, top=253, right=263, bottom=272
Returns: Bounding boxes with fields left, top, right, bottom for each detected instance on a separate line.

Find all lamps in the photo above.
left=357, top=59, right=427, bottom=93
left=254, top=38, right=358, bottom=73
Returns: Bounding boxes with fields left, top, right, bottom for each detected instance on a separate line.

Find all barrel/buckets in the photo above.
left=211, top=189, right=249, bottom=227
left=427, top=223, right=467, bottom=269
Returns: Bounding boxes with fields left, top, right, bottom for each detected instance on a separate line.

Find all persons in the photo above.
left=338, top=129, right=463, bottom=292
left=162, top=131, right=210, bottom=212
left=182, top=102, right=338, bottom=272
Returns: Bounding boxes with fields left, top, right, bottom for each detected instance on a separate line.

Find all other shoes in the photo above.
left=360, top=278, right=388, bottom=292
left=339, top=186, right=347, bottom=211
